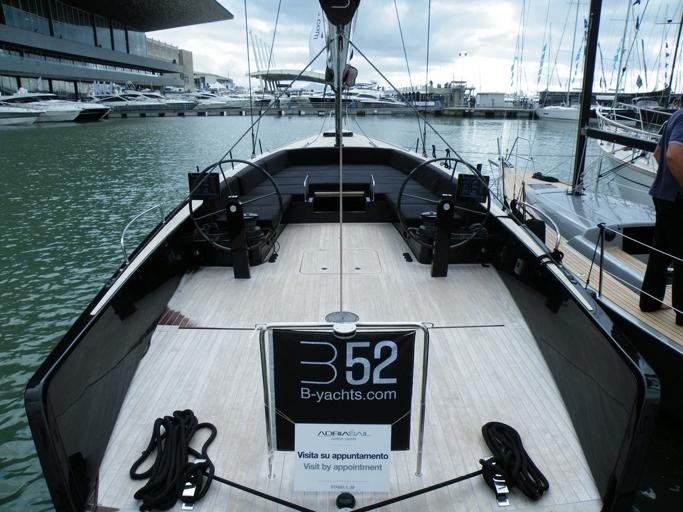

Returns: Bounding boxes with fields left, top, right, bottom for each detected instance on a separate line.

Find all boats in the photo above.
left=0, top=87, right=110, bottom=124
left=94, top=91, right=199, bottom=114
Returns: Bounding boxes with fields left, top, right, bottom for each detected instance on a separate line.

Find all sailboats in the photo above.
left=190, top=32, right=475, bottom=116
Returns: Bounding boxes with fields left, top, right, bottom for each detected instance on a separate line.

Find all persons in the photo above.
left=636, top=107, right=682, bottom=325
